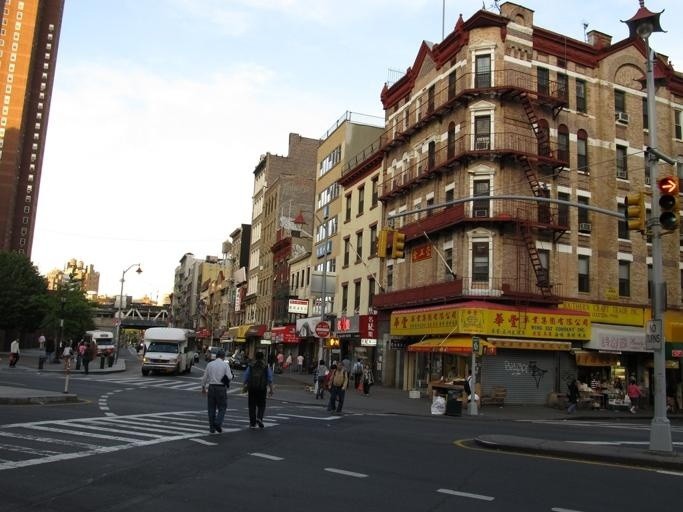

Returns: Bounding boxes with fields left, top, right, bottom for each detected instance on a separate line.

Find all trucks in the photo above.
left=139, top=324, right=196, bottom=378
left=84, top=330, right=115, bottom=357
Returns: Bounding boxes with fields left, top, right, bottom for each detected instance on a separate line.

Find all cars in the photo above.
left=205, top=346, right=221, bottom=360
left=228, top=353, right=251, bottom=368
left=194, top=352, right=200, bottom=361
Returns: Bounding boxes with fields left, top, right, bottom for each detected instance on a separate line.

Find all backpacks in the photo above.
left=356, top=363, right=362, bottom=375
left=249, top=359, right=268, bottom=389
left=464, top=377, right=471, bottom=394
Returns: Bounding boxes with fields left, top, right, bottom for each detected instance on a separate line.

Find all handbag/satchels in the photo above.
left=70, top=349, right=73, bottom=355
left=325, top=370, right=329, bottom=375
left=625, top=395, right=631, bottom=403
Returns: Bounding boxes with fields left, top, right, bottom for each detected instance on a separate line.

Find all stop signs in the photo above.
left=314, top=320, right=330, bottom=337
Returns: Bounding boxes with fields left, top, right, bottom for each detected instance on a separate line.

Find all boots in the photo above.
left=630, top=407, right=636, bottom=413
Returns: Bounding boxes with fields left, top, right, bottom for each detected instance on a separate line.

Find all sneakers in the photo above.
left=214, top=423, right=222, bottom=433
left=250, top=425, right=255, bottom=429
left=256, top=418, right=264, bottom=427
left=209, top=430, right=215, bottom=432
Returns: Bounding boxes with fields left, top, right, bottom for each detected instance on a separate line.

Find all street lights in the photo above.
left=618, top=0, right=676, bottom=454
left=293, top=206, right=329, bottom=391
left=52, top=290, right=68, bottom=363
left=114, top=262, right=142, bottom=363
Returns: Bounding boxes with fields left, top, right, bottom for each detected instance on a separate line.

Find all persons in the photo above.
left=613, top=377, right=623, bottom=391
left=313, top=356, right=375, bottom=413
left=240, top=351, right=273, bottom=431
left=7, top=332, right=96, bottom=374
left=464, top=369, right=479, bottom=403
left=275, top=351, right=284, bottom=369
left=447, top=367, right=457, bottom=381
left=295, top=352, right=304, bottom=372
left=285, top=353, right=293, bottom=371
left=626, top=379, right=640, bottom=414
left=566, top=378, right=581, bottom=414
left=200, top=349, right=233, bottom=433
left=590, top=377, right=599, bottom=391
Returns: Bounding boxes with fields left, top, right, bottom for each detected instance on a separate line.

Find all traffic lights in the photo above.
left=656, top=173, right=679, bottom=231
left=620, top=188, right=648, bottom=233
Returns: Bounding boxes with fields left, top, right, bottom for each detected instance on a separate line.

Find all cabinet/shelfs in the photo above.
left=428, top=380, right=481, bottom=405
